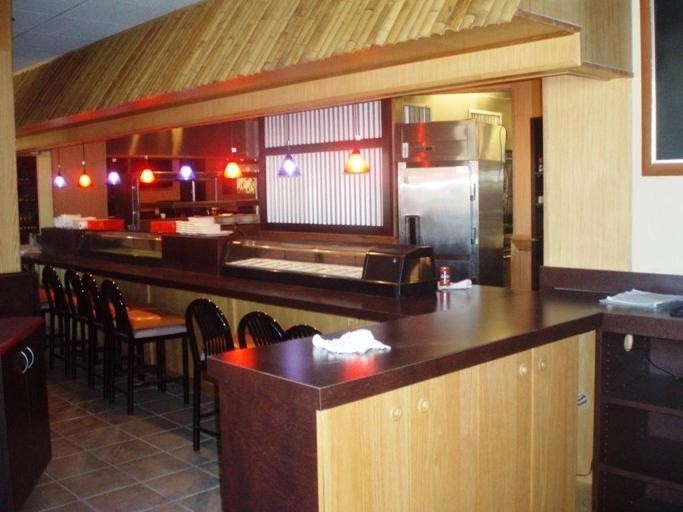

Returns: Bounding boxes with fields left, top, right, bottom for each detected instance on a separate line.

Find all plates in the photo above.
left=53, top=212, right=126, bottom=232
left=148, top=215, right=234, bottom=238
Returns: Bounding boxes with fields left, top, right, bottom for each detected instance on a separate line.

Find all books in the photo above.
left=599, top=288, right=683, bottom=308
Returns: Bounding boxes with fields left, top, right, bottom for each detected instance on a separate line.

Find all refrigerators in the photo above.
left=394, top=116, right=506, bottom=291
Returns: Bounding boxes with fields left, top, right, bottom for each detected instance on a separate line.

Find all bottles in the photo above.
left=439, top=264, right=450, bottom=286
left=211, top=207, right=218, bottom=216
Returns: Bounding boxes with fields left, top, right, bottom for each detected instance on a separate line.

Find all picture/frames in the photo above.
left=640, top=0, right=683, bottom=175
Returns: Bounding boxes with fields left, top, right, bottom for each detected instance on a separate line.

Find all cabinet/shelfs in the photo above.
left=131, top=167, right=260, bottom=231
left=0, top=316, right=53, bottom=512
left=592, top=313, right=683, bottom=512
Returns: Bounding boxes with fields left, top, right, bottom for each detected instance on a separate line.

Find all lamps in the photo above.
left=175, top=128, right=196, bottom=182
left=139, top=137, right=155, bottom=183
left=344, top=102, right=370, bottom=176
left=222, top=123, right=242, bottom=179
left=104, top=139, right=122, bottom=186
left=51, top=147, right=69, bottom=189
left=77, top=144, right=93, bottom=189
left=278, top=113, right=301, bottom=178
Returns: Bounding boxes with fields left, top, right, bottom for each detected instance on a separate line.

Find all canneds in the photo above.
left=440, top=266, right=451, bottom=286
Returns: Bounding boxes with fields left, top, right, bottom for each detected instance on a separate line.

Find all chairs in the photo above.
left=312, top=329, right=392, bottom=354
